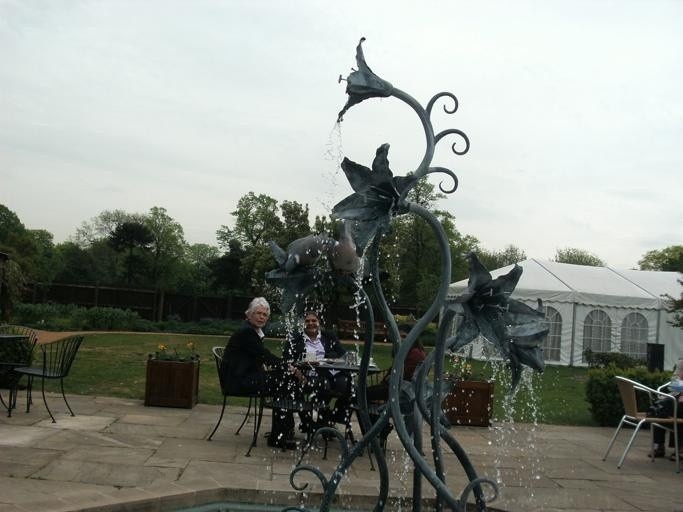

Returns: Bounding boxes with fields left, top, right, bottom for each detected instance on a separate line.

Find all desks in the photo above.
left=0, top=334, right=31, bottom=410
left=291, top=360, right=380, bottom=460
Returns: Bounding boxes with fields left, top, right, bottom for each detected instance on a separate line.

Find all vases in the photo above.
left=144, top=358, right=200, bottom=410
left=440, top=380, right=494, bottom=428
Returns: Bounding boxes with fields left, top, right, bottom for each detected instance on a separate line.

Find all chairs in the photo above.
left=206, top=346, right=267, bottom=457
left=601, top=375, right=683, bottom=474
left=365, top=364, right=434, bottom=471
left=0, top=325, right=39, bottom=409
left=7, top=334, right=86, bottom=423
left=243, top=358, right=329, bottom=467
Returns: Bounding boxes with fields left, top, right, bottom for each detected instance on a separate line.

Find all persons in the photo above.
left=282, top=311, right=358, bottom=433
left=647, top=360, right=683, bottom=462
left=220, top=297, right=307, bottom=450
left=358, top=324, right=432, bottom=453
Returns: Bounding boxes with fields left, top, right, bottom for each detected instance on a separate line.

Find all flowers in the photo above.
left=448, top=353, right=473, bottom=381
left=148, top=341, right=200, bottom=362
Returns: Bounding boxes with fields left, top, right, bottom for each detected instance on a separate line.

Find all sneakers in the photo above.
left=267, top=432, right=298, bottom=450
